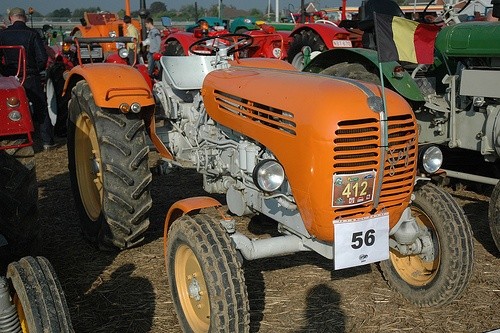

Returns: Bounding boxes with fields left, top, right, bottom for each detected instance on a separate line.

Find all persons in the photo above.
left=143, top=18, right=163, bottom=75
left=0, top=9, right=54, bottom=151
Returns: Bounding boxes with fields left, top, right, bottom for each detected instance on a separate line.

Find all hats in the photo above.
left=8, top=6, right=26, bottom=20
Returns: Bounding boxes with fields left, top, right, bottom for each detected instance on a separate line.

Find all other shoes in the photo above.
left=43, top=143, right=60, bottom=150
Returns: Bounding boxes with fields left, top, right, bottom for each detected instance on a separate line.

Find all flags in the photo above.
left=373, top=11, right=436, bottom=64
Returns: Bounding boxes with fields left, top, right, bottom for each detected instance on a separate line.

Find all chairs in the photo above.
left=158, top=54, right=233, bottom=103
left=337, top=19, right=361, bottom=31
left=357, top=1, right=430, bottom=73
